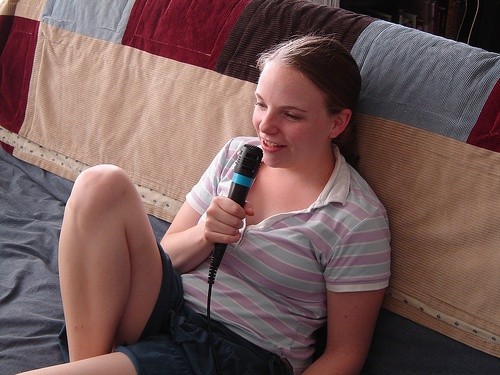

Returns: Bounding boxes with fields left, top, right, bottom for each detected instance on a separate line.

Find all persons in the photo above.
left=16, top=34, right=392, bottom=375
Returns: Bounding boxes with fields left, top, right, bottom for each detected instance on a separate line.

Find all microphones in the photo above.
left=208, top=143, right=263, bottom=283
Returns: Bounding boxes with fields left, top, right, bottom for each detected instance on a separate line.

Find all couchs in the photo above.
left=0, top=0, right=499, bottom=375
